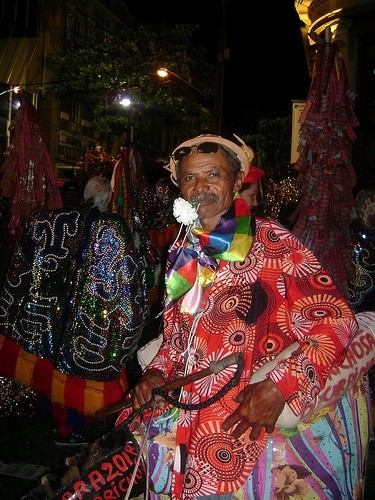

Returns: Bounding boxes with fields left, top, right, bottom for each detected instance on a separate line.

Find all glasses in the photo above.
left=170, top=142, right=240, bottom=171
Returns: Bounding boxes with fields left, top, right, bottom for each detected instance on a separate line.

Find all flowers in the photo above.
left=173, top=197, right=200, bottom=225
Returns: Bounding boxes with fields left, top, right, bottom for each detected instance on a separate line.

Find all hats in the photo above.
left=244, top=166, right=264, bottom=184
left=170, top=130, right=254, bottom=179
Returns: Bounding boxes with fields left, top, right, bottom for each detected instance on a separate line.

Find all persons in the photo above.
left=0, top=177, right=154, bottom=447
left=239, top=165, right=266, bottom=211
left=117, top=133, right=370, bottom=500
